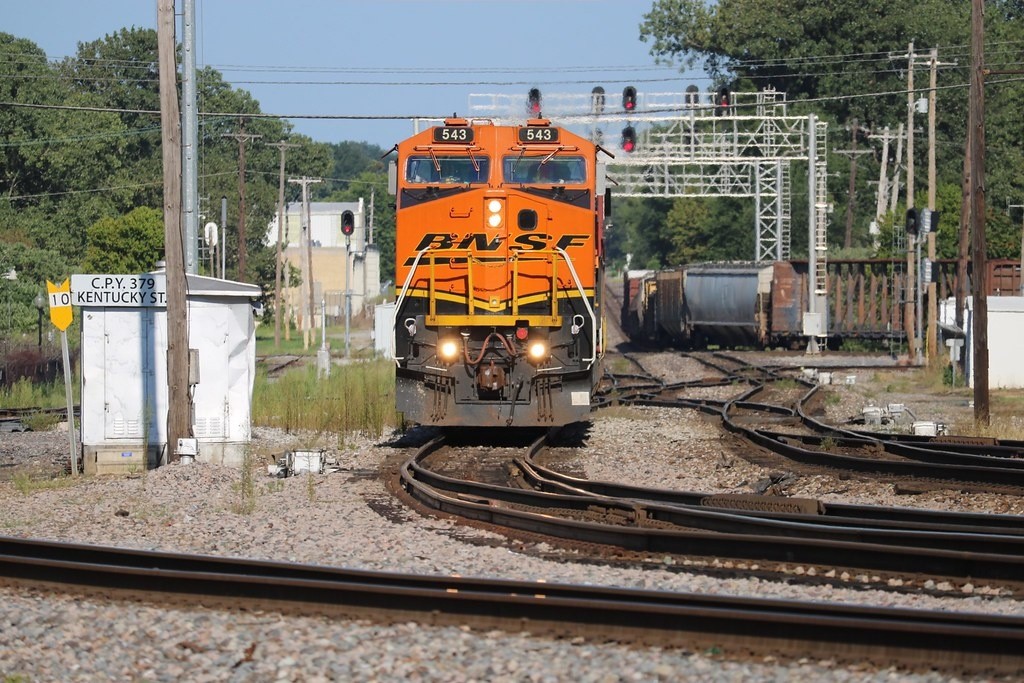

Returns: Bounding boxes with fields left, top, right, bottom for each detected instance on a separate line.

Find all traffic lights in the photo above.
left=622, top=86, right=637, bottom=111
left=341, top=210, right=355, bottom=235
left=717, top=84, right=730, bottom=111
left=621, top=126, right=637, bottom=154
left=527, top=88, right=542, bottom=115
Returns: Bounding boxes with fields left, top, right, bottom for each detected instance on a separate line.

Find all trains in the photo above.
left=381, top=110, right=619, bottom=429
left=619, top=261, right=846, bottom=351
